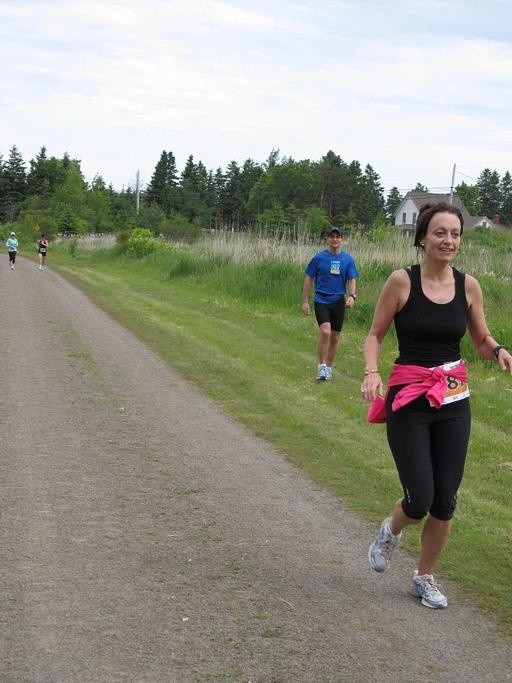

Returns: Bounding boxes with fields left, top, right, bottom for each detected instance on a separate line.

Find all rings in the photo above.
left=364, top=389, right=367, bottom=390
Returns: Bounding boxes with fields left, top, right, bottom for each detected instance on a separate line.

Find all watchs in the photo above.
left=350, top=294, right=356, bottom=300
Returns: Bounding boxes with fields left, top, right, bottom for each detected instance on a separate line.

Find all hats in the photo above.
left=327, top=226, right=341, bottom=236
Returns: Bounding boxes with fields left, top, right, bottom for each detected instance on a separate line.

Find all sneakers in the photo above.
left=409, top=570, right=448, bottom=608
left=317, top=364, right=327, bottom=379
left=325, top=366, right=333, bottom=380
left=368, top=516, right=403, bottom=573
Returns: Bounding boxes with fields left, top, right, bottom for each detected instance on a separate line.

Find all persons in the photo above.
left=302, top=228, right=358, bottom=382
left=6, top=232, right=18, bottom=269
left=37, top=233, right=48, bottom=271
left=360, top=202, right=512, bottom=609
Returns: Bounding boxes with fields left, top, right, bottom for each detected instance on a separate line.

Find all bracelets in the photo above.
left=364, top=370, right=379, bottom=375
left=491, top=346, right=508, bottom=359
